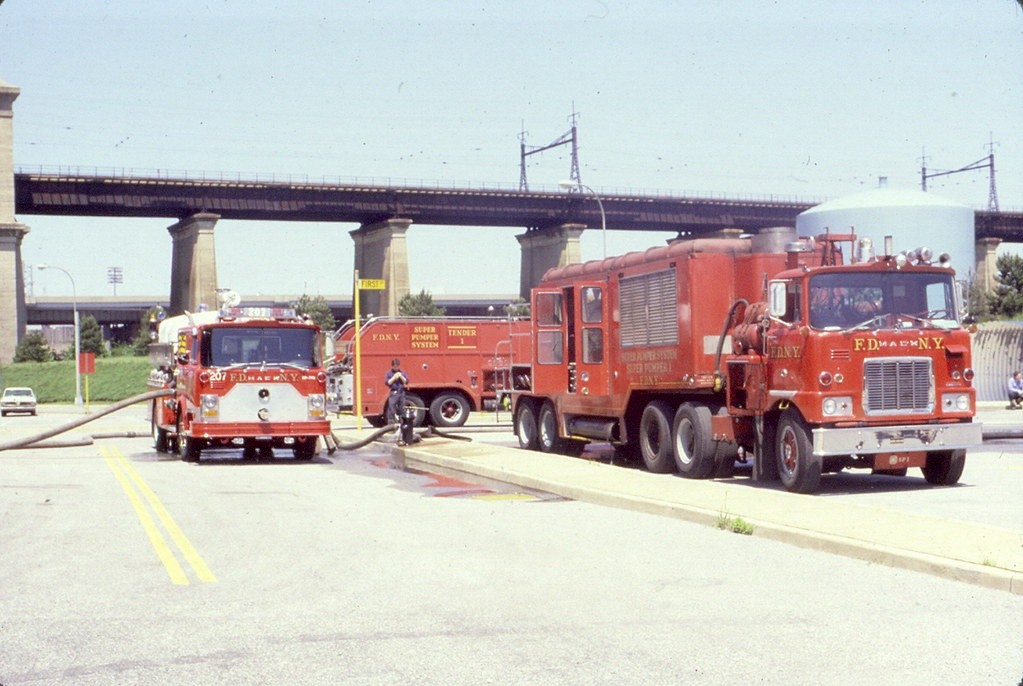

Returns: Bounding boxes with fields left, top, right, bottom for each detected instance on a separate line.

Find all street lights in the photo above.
left=559, top=179, right=607, bottom=259
left=37, top=263, right=83, bottom=405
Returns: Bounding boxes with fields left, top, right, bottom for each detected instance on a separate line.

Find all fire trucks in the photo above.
left=147, top=306, right=330, bottom=461
left=326, top=314, right=531, bottom=428
left=495, top=226, right=982, bottom=493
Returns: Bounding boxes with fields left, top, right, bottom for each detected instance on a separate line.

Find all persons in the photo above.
left=1006, top=371, right=1023, bottom=408
left=385, top=359, right=409, bottom=434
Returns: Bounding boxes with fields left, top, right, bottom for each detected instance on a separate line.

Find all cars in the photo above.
left=0, top=387, right=37, bottom=417
